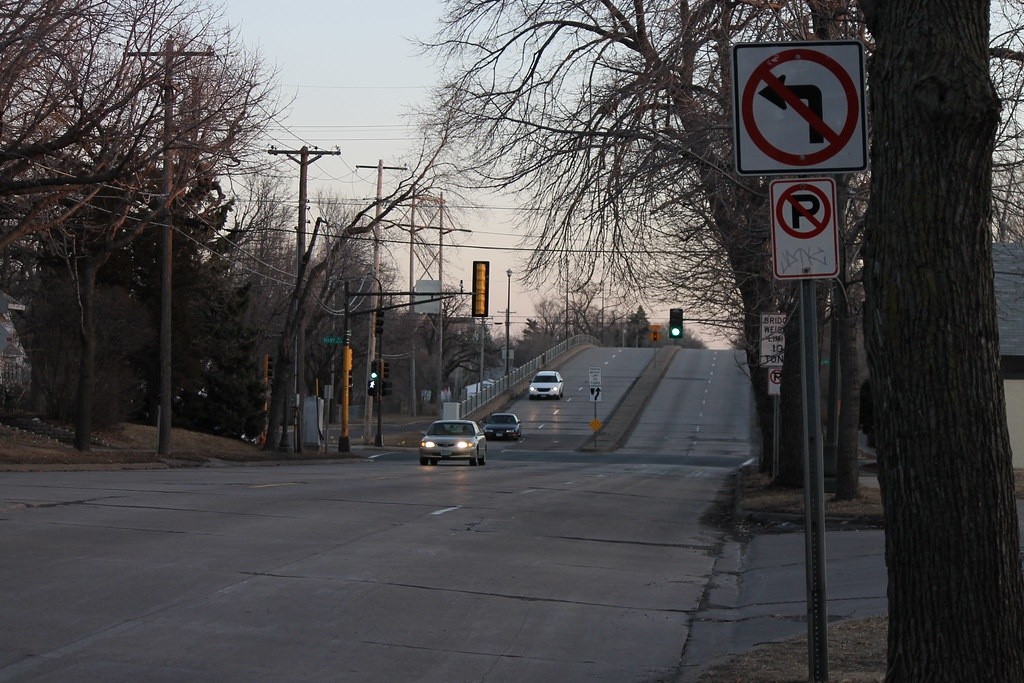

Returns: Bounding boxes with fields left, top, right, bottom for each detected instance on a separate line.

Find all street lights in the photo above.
left=337, top=275, right=366, bottom=451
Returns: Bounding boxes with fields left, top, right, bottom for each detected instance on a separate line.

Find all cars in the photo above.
left=419, top=419, right=487, bottom=466
left=529, top=370, right=564, bottom=400
left=484, top=412, right=523, bottom=440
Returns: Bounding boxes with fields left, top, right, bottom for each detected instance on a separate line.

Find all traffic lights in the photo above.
left=669, top=308, right=684, bottom=339
left=370, top=358, right=379, bottom=379
left=375, top=306, right=385, bottom=337
left=265, top=354, right=273, bottom=377
left=368, top=380, right=378, bottom=396
left=379, top=380, right=393, bottom=397
left=381, top=360, right=390, bottom=382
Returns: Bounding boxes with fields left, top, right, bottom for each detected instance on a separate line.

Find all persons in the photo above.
left=462, top=426, right=470, bottom=435
left=506, top=417, right=510, bottom=422
left=437, top=426, right=446, bottom=434
